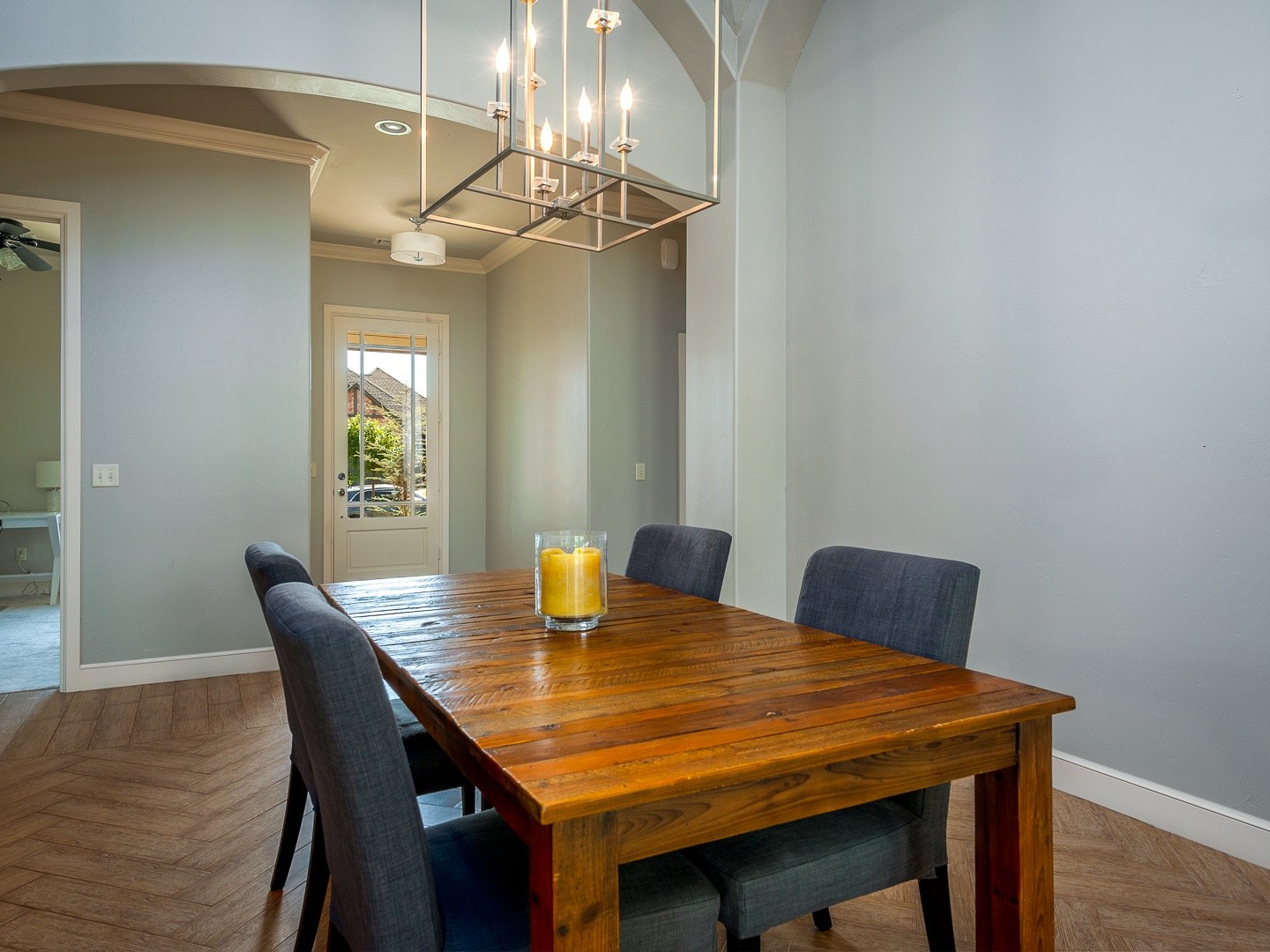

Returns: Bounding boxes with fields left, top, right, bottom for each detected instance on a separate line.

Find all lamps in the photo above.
left=390, top=217, right=446, bottom=265
left=422, top=0, right=718, bottom=253
left=35, top=461, right=61, bottom=512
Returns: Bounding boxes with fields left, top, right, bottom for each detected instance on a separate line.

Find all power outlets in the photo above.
left=16, top=547, right=27, bottom=561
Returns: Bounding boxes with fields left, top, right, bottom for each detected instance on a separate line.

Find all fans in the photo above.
left=0, top=218, right=61, bottom=273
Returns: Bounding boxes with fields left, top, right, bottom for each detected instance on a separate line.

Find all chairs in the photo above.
left=264, top=581, right=720, bottom=952
left=624, top=523, right=732, bottom=601
left=688, top=544, right=981, bottom=952
left=244, top=541, right=492, bottom=952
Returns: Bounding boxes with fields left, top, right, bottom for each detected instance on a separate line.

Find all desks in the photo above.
left=0, top=511, right=61, bottom=606
left=318, top=570, right=1081, bottom=952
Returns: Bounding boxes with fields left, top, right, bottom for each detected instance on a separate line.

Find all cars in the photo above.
left=348, top=484, right=427, bottom=518
